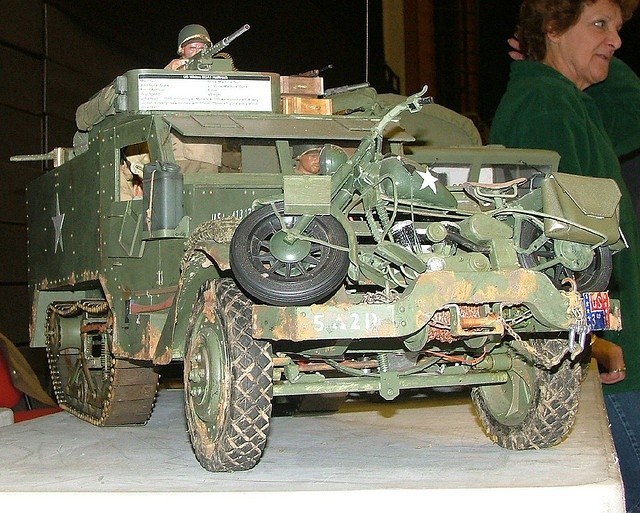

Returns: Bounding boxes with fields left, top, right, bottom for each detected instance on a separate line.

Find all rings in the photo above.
left=610, top=366, right=618, bottom=375
left=620, top=366, right=628, bottom=375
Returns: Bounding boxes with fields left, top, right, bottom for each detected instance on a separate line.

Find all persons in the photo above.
left=163, top=22, right=237, bottom=72
left=288, top=141, right=323, bottom=174
left=487, top=1, right=640, bottom=509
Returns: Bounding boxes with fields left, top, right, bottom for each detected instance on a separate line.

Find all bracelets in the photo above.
left=588, top=333, right=599, bottom=347
